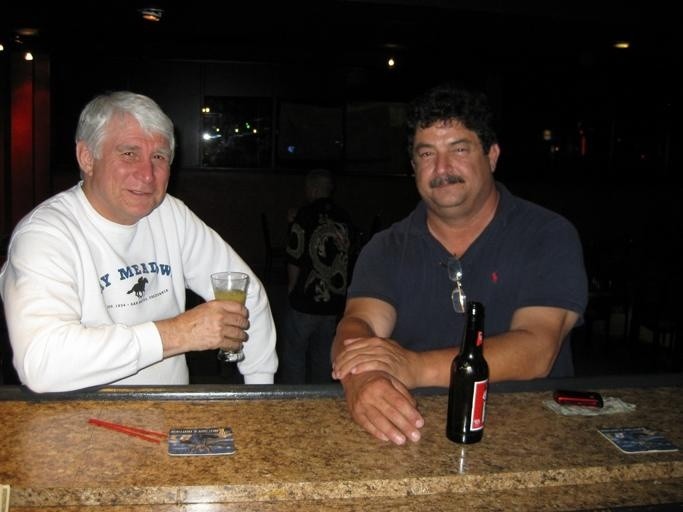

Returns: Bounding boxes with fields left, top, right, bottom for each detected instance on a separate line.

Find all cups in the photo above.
left=210, top=272, right=249, bottom=362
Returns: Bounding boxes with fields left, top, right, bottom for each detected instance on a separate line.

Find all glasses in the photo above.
left=445, top=255, right=470, bottom=315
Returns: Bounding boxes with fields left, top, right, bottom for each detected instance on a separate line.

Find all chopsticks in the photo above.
left=87, top=417, right=167, bottom=445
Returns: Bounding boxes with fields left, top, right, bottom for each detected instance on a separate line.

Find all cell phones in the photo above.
left=552, top=389, right=604, bottom=408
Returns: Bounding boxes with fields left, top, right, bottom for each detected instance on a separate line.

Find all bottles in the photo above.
left=445, top=301, right=489, bottom=445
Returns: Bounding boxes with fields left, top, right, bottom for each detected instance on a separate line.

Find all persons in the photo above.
left=275, top=170, right=354, bottom=384
left=0, top=91, right=280, bottom=394
left=329, top=84, right=588, bottom=447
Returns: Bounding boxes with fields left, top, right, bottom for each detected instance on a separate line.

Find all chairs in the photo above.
left=258, top=211, right=292, bottom=286
